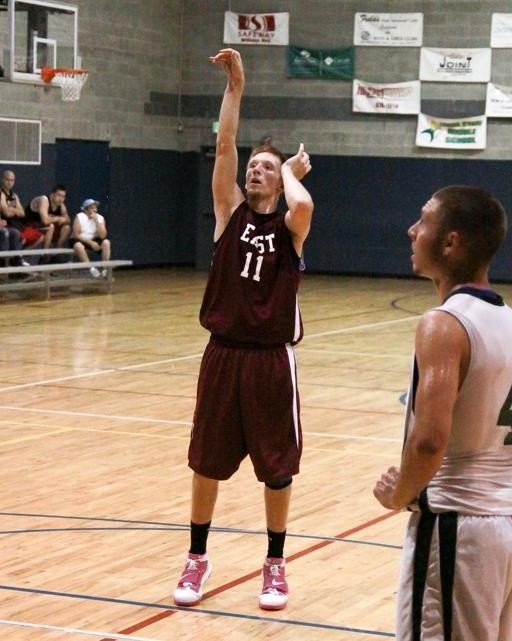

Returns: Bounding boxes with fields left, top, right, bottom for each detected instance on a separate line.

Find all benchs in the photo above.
left=0, top=243, right=134, bottom=301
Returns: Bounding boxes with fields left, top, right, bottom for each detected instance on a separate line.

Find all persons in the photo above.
left=371, top=185, right=509, bottom=641
left=174, top=48, right=316, bottom=613
left=1, top=168, right=32, bottom=272
left=71, top=199, right=116, bottom=282
left=25, top=184, right=73, bottom=263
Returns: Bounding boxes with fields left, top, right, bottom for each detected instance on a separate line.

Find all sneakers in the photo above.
left=90, top=267, right=107, bottom=279
left=39, top=255, right=68, bottom=264
left=174, top=552, right=213, bottom=607
left=19, top=258, right=30, bottom=267
left=259, top=555, right=289, bottom=611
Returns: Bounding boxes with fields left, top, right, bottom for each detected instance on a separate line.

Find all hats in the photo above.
left=81, top=198, right=100, bottom=211
